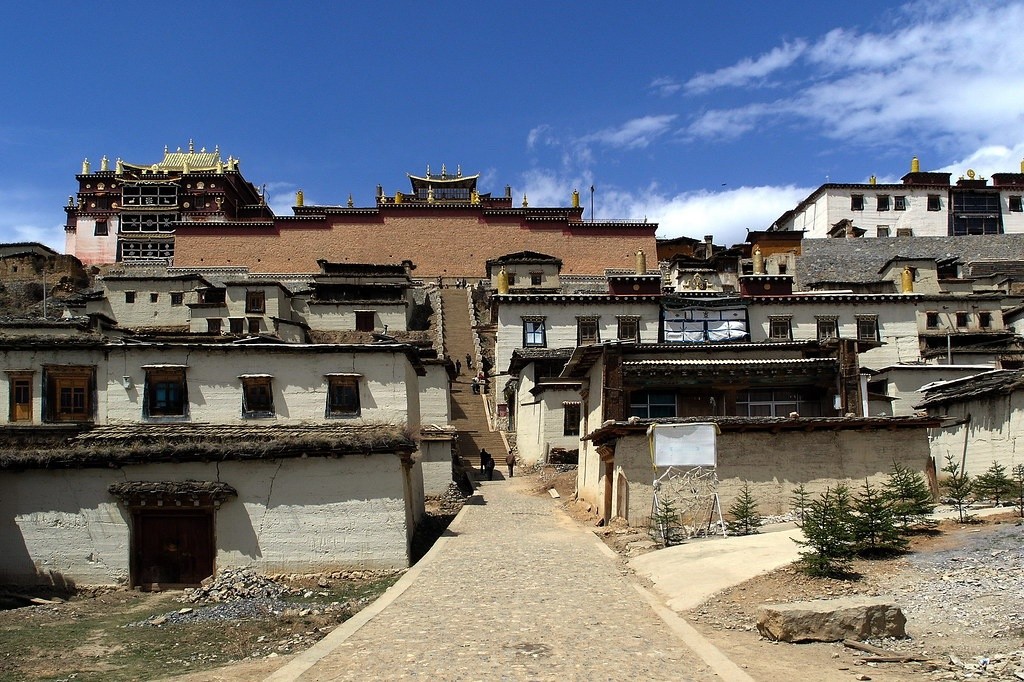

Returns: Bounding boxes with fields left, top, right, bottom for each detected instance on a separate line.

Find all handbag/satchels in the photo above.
left=470, top=361, right=472, bottom=365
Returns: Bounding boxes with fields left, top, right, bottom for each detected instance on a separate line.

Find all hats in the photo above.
left=471, top=378, right=475, bottom=379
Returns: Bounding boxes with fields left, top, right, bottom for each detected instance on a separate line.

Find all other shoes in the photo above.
left=480, top=473, right=482, bottom=475
left=509, top=476, right=511, bottom=478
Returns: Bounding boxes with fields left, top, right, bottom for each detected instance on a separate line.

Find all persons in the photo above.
left=446, top=355, right=461, bottom=381
left=471, top=374, right=484, bottom=394
left=480, top=448, right=495, bottom=481
left=466, top=352, right=471, bottom=368
left=506, top=450, right=516, bottom=478
left=437, top=276, right=466, bottom=289
